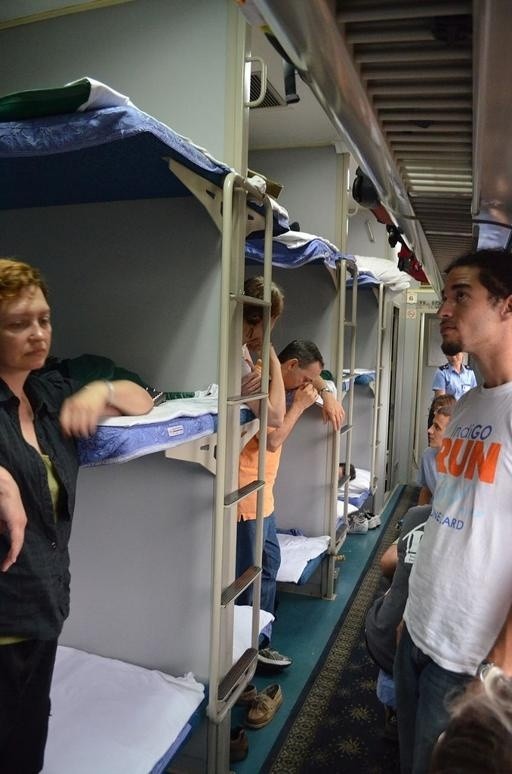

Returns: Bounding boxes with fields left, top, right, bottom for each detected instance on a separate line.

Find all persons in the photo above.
left=425, top=661, right=510, bottom=774
left=240, top=337, right=349, bottom=673
left=242, top=272, right=289, bottom=428
left=361, top=341, right=478, bottom=676
left=386, top=248, right=510, bottom=771
left=1, top=254, right=161, bottom=773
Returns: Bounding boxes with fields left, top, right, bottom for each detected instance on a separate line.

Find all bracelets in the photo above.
left=102, top=376, right=112, bottom=406
left=317, top=383, right=335, bottom=399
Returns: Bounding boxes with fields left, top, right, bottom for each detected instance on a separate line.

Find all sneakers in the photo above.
left=256, top=647, right=293, bottom=669
left=349, top=510, right=381, bottom=535
left=230, top=728, right=249, bottom=761
left=234, top=684, right=284, bottom=728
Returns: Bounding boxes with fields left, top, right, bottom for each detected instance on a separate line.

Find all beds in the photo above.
left=0, top=70, right=414, bottom=773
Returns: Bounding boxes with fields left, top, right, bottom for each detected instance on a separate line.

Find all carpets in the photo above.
left=257, top=485, right=427, bottom=773
